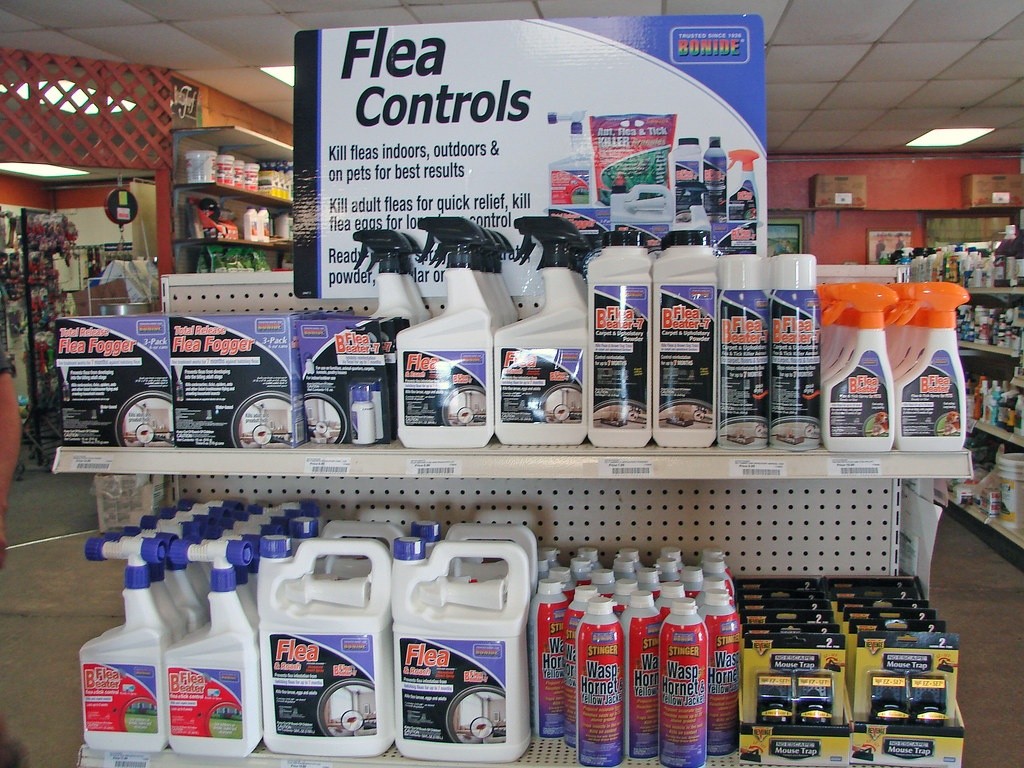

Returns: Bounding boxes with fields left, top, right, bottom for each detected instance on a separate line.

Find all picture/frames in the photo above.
left=768, top=216, right=803, bottom=257
left=865, top=227, right=913, bottom=265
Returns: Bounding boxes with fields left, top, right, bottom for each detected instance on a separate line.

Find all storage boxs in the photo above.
left=960, top=173, right=1024, bottom=210
left=733, top=574, right=965, bottom=768
left=70, top=278, right=130, bottom=317
left=808, top=173, right=867, bottom=210
left=186, top=197, right=239, bottom=239
left=948, top=436, right=1002, bottom=517
left=95, top=474, right=168, bottom=533
left=52, top=311, right=311, bottom=449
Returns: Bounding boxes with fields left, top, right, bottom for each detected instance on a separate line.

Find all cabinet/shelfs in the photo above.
left=50, top=264, right=974, bottom=768
left=933, top=287, right=1024, bottom=574
left=172, top=126, right=293, bottom=273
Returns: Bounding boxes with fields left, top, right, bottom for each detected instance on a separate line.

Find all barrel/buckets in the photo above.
left=185, top=151, right=217, bottom=184
left=216, top=155, right=235, bottom=186
left=234, top=160, right=245, bottom=189
left=998, top=453, right=1024, bottom=529
left=245, top=163, right=260, bottom=192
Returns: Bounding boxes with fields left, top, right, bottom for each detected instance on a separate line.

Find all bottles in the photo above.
left=956, top=307, right=1024, bottom=351
left=79, top=499, right=538, bottom=762
left=963, top=367, right=1024, bottom=437
left=528, top=545, right=662, bottom=767
left=704, top=137, right=727, bottom=224
left=655, top=546, right=741, bottom=768
left=718, top=253, right=821, bottom=451
left=244, top=207, right=258, bottom=241
left=258, top=207, right=270, bottom=243
left=669, top=138, right=701, bottom=215
left=351, top=385, right=376, bottom=445
left=270, top=211, right=293, bottom=240
left=966, top=436, right=997, bottom=463
left=875, top=209, right=1024, bottom=289
left=588, top=232, right=716, bottom=447
left=258, top=161, right=294, bottom=201
